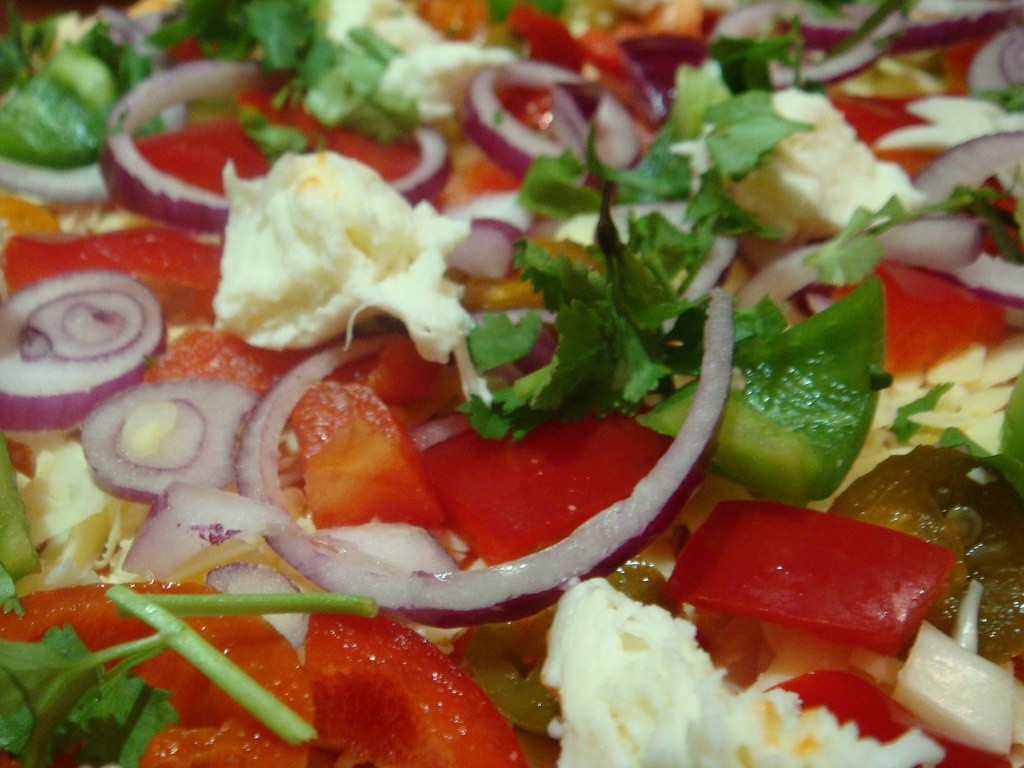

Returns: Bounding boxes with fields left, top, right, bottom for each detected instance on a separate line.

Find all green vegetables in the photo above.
left=0, top=0, right=1024, bottom=768
